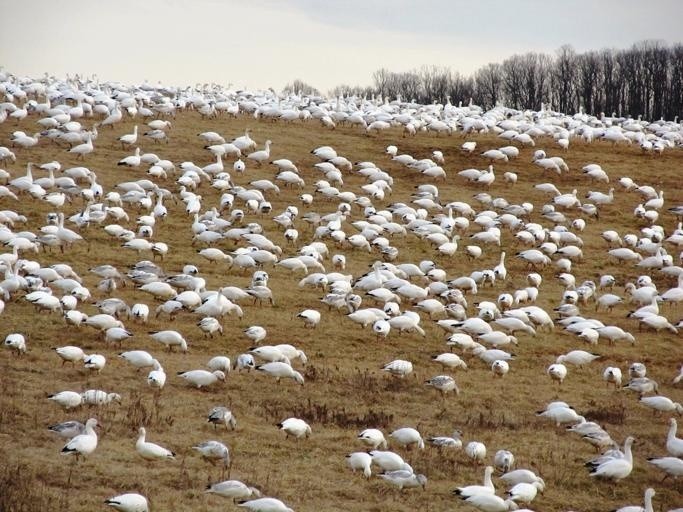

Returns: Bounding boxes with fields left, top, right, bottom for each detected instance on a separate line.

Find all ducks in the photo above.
left=0, top=72, right=683, bottom=512
